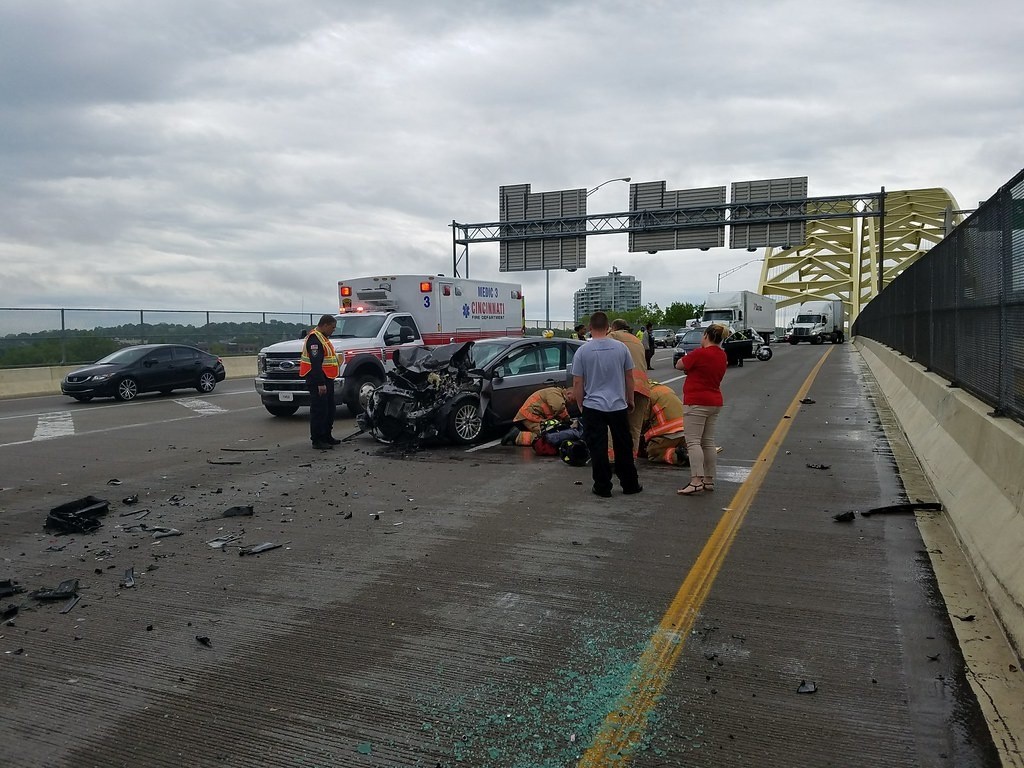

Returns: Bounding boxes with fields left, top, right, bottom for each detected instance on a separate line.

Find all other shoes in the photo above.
left=312, top=441, right=332, bottom=449
left=325, top=435, right=340, bottom=445
left=501, top=426, right=519, bottom=446
left=675, top=447, right=690, bottom=466
left=647, top=366, right=653, bottom=370
left=623, top=484, right=643, bottom=495
left=592, top=487, right=611, bottom=497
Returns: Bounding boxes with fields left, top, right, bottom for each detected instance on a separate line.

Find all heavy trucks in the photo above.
left=696, top=289, right=776, bottom=345
left=786, top=299, right=846, bottom=344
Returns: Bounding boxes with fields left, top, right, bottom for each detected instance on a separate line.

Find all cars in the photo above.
left=769, top=334, right=777, bottom=342
left=357, top=334, right=587, bottom=444
left=784, top=328, right=792, bottom=341
left=673, top=326, right=753, bottom=369
left=59, top=344, right=226, bottom=402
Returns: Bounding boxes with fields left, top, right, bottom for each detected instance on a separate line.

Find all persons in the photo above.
left=675, top=324, right=729, bottom=495
left=299, top=315, right=341, bottom=450
left=636, top=323, right=655, bottom=370
left=501, top=387, right=575, bottom=446
left=569, top=325, right=587, bottom=341
left=570, top=311, right=643, bottom=498
left=644, top=379, right=684, bottom=465
left=606, top=319, right=651, bottom=463
left=729, top=331, right=747, bottom=367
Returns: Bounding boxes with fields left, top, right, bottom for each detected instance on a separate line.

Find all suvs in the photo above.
left=652, top=329, right=676, bottom=348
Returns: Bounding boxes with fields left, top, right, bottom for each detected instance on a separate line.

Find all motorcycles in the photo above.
left=748, top=326, right=773, bottom=362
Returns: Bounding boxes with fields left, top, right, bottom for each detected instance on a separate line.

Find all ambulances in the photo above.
left=254, top=274, right=525, bottom=417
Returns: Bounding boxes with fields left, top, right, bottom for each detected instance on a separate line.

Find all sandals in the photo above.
left=701, top=479, right=715, bottom=492
left=678, top=481, right=704, bottom=495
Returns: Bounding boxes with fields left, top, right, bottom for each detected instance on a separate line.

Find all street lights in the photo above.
left=608, top=267, right=622, bottom=310
left=546, top=177, right=630, bottom=328
